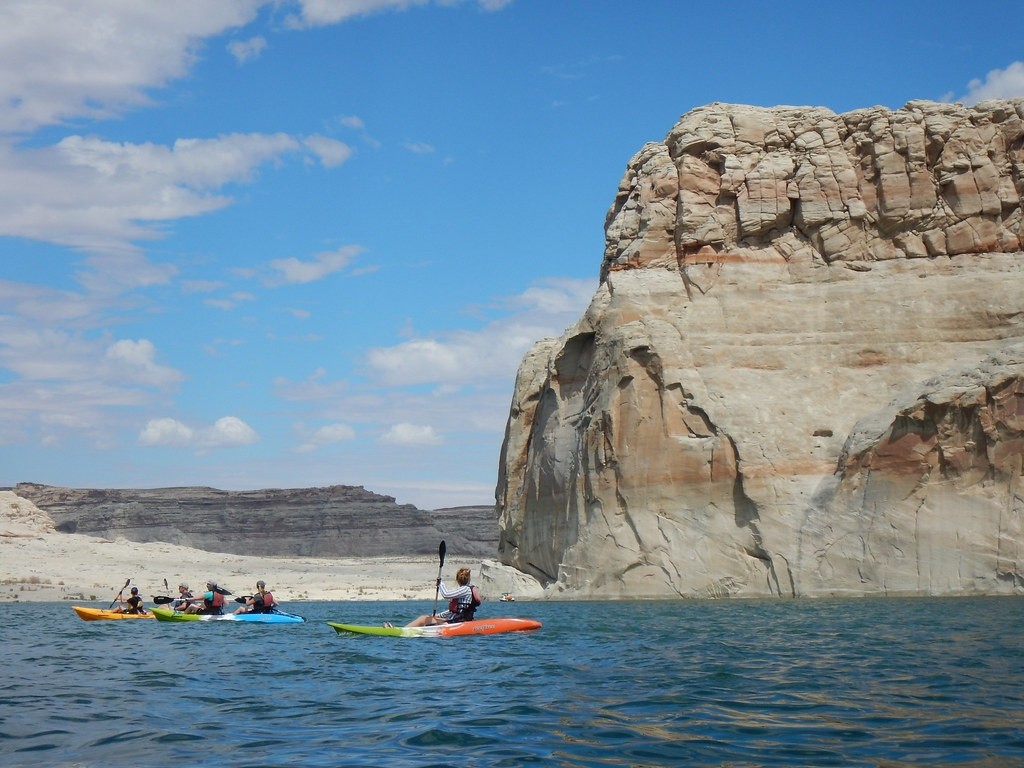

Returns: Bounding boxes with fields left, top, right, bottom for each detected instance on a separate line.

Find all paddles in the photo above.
left=110, top=578, right=131, bottom=608
left=163, top=578, right=172, bottom=610
left=153, top=586, right=254, bottom=605
left=215, top=586, right=308, bottom=622
left=432, top=539, right=447, bottom=617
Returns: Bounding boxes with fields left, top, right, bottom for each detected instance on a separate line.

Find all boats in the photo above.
left=499, top=593, right=515, bottom=602
left=71, top=606, right=156, bottom=621
left=148, top=607, right=307, bottom=622
left=327, top=618, right=543, bottom=638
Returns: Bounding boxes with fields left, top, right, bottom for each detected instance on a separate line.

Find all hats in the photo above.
left=257, top=581, right=267, bottom=587
left=178, top=583, right=189, bottom=589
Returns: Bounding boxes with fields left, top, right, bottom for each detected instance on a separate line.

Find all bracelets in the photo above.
left=174, top=607, right=175, bottom=610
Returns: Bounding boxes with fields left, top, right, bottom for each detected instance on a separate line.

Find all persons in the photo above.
left=185, top=580, right=229, bottom=614
left=383, top=568, right=476, bottom=628
left=101, top=587, right=144, bottom=614
left=146, top=583, right=195, bottom=615
left=235, top=580, right=278, bottom=614
left=502, top=594, right=506, bottom=599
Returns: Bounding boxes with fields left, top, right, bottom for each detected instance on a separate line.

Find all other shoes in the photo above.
left=383, top=622, right=389, bottom=628
left=387, top=622, right=393, bottom=628
